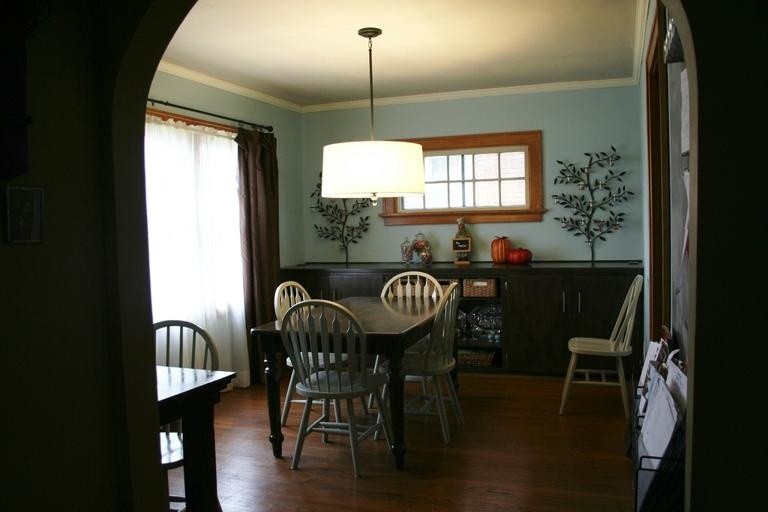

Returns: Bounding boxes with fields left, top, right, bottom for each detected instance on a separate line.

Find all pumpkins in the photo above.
left=491, top=239, right=511, bottom=263
left=507, top=249, right=532, bottom=263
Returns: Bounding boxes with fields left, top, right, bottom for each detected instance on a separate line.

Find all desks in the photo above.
left=155, top=363, right=238, bottom=511
left=250, top=293, right=440, bottom=471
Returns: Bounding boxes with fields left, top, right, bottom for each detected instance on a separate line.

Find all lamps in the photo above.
left=318, top=28, right=427, bottom=207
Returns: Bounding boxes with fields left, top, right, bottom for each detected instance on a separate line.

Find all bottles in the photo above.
left=400, top=232, right=433, bottom=265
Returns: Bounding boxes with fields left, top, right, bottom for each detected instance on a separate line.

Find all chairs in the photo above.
left=368, top=271, right=465, bottom=445
left=274, top=281, right=394, bottom=478
left=153, top=319, right=220, bottom=371
left=558, top=274, right=643, bottom=419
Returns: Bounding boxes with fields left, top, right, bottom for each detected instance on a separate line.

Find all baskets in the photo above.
left=393, top=279, right=424, bottom=296
left=457, top=351, right=494, bottom=366
left=428, top=279, right=461, bottom=296
left=463, top=279, right=496, bottom=297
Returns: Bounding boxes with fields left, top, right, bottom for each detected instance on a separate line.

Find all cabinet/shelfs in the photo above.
left=388, top=261, right=500, bottom=373
left=500, top=259, right=644, bottom=375
left=280, top=261, right=388, bottom=305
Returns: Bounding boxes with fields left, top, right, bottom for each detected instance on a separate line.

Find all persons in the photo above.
left=455, top=223, right=472, bottom=260
